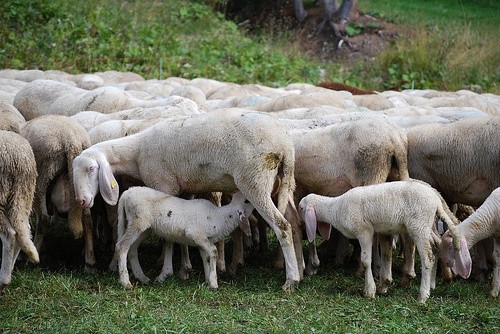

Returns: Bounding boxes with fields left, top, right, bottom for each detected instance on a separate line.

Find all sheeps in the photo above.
left=0, top=69, right=500, bottom=304
